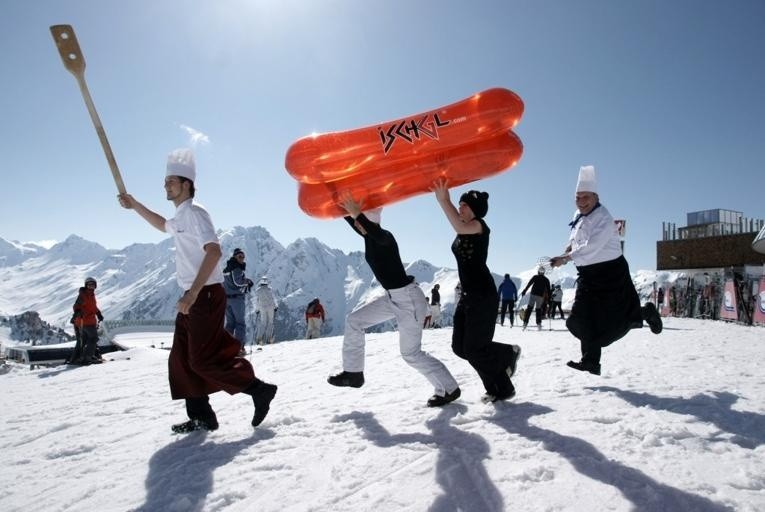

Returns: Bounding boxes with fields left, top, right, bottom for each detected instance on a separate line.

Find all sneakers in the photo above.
left=426, top=388, right=461, bottom=407
left=481, top=388, right=515, bottom=403
left=506, top=344, right=521, bottom=377
left=327, top=371, right=364, bottom=388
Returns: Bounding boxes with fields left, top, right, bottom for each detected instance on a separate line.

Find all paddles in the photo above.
left=49, top=25, right=128, bottom=197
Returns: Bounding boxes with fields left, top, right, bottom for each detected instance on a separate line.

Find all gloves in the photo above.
left=96, top=311, right=104, bottom=322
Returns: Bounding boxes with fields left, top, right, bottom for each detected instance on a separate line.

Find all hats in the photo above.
left=459, top=191, right=489, bottom=216
left=363, top=207, right=383, bottom=223
left=261, top=277, right=268, bottom=285
left=575, top=165, right=596, bottom=193
left=314, top=297, right=320, bottom=304
left=233, top=248, right=244, bottom=254
left=85, top=278, right=97, bottom=288
left=165, top=148, right=195, bottom=182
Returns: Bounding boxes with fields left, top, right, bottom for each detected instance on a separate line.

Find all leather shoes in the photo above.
left=252, top=384, right=277, bottom=426
left=644, top=302, right=662, bottom=334
left=172, top=413, right=218, bottom=433
left=567, top=359, right=601, bottom=375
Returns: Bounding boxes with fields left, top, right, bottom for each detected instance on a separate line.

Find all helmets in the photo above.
left=538, top=267, right=545, bottom=275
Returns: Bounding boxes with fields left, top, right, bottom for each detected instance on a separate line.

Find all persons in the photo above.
left=302, top=296, right=325, bottom=339
left=668, top=286, right=677, bottom=317
left=546, top=192, right=664, bottom=378
left=329, top=187, right=461, bottom=412
left=428, top=176, right=524, bottom=405
left=67, top=275, right=104, bottom=363
left=252, top=275, right=278, bottom=345
left=116, top=177, right=278, bottom=434
left=220, top=249, right=251, bottom=356
left=657, top=288, right=664, bottom=312
left=417, top=266, right=567, bottom=332
